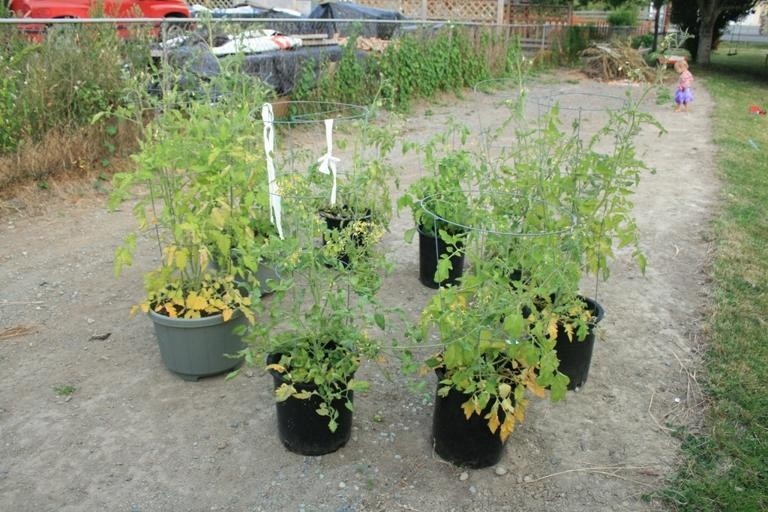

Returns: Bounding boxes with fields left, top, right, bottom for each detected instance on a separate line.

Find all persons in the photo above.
left=672, top=59, right=697, bottom=112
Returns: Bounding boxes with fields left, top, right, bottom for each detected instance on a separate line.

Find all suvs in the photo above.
left=1, top=0, right=192, bottom=44
